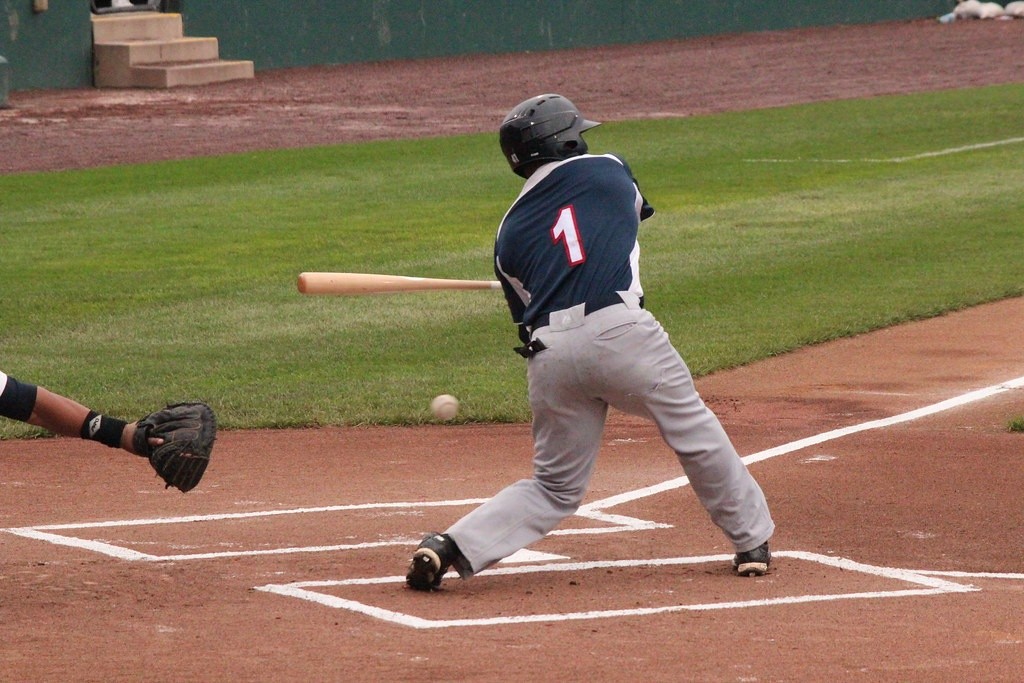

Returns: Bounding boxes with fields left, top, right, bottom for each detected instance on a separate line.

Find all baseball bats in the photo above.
left=296, top=271, right=504, bottom=296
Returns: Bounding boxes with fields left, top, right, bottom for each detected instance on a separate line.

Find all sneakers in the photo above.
left=732, top=540, right=772, bottom=574
left=405, top=530, right=455, bottom=590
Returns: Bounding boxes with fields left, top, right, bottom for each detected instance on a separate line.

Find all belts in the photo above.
left=530, top=293, right=623, bottom=336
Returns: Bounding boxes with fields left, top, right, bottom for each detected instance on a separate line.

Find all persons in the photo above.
left=406, top=93, right=774, bottom=590
left=0, top=371, right=217, bottom=493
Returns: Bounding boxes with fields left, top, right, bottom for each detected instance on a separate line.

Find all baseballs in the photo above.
left=429, top=393, right=460, bottom=422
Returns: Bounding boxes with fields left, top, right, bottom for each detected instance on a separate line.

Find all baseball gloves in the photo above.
left=130, top=401, right=219, bottom=496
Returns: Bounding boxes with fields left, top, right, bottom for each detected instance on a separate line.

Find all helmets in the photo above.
left=499, top=93, right=603, bottom=179
